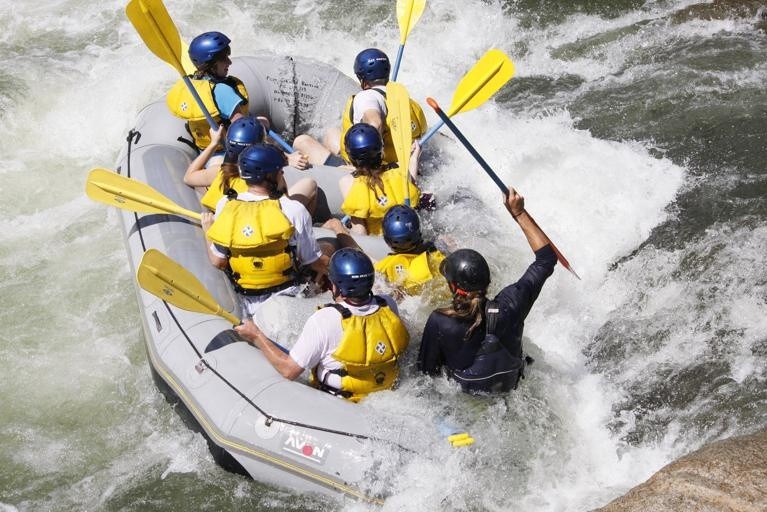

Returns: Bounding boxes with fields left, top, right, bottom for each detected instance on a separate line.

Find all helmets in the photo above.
left=327, top=248, right=374, bottom=297
left=227, top=118, right=263, bottom=152
left=439, top=249, right=489, bottom=296
left=189, top=32, right=231, bottom=69
left=355, top=49, right=390, bottom=80
left=344, top=123, right=384, bottom=158
left=238, top=144, right=289, bottom=183
left=383, top=205, right=421, bottom=252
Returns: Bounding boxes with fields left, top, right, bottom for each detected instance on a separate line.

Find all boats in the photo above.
left=115, top=54, right=536, bottom=508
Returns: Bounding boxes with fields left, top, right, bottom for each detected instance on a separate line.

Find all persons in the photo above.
left=232, top=247, right=410, bottom=403
left=184, top=116, right=319, bottom=227
left=293, top=48, right=426, bottom=167
left=322, top=204, right=455, bottom=305
left=166, top=31, right=309, bottom=170
left=416, top=185, right=557, bottom=396
left=340, top=124, right=423, bottom=240
left=202, top=142, right=335, bottom=305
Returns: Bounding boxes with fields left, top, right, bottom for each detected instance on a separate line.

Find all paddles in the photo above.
left=125, top=0, right=226, bottom=147
left=423, top=96, right=580, bottom=280
left=391, top=0, right=427, bottom=82
left=418, top=48, right=515, bottom=149
left=137, top=249, right=290, bottom=354
left=85, top=167, right=203, bottom=221
